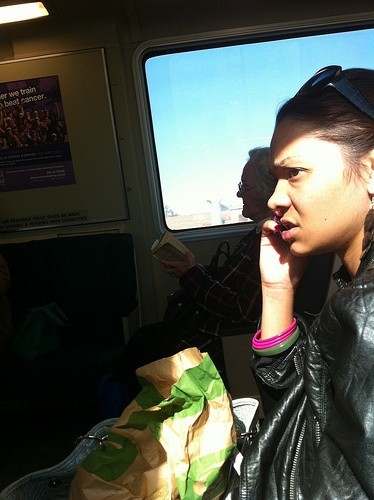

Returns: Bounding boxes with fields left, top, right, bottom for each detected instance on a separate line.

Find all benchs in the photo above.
left=0, top=229, right=141, bottom=436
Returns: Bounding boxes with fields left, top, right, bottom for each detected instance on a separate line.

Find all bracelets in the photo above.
left=251, top=318, right=300, bottom=358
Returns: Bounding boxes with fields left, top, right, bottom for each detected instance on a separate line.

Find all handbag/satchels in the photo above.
left=164, top=239, right=231, bottom=325
left=0, top=398, right=261, bottom=500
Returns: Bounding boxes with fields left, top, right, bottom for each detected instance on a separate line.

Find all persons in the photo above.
left=116, top=150, right=311, bottom=419
left=222, top=67, right=374, bottom=500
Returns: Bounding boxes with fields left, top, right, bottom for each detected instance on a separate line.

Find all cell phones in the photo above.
left=272, top=214, right=287, bottom=235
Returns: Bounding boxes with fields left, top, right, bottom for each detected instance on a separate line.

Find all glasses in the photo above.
left=238, top=182, right=261, bottom=193
left=294, top=65, right=374, bottom=128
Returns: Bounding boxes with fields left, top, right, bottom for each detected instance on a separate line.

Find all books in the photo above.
left=149, top=232, right=195, bottom=268
